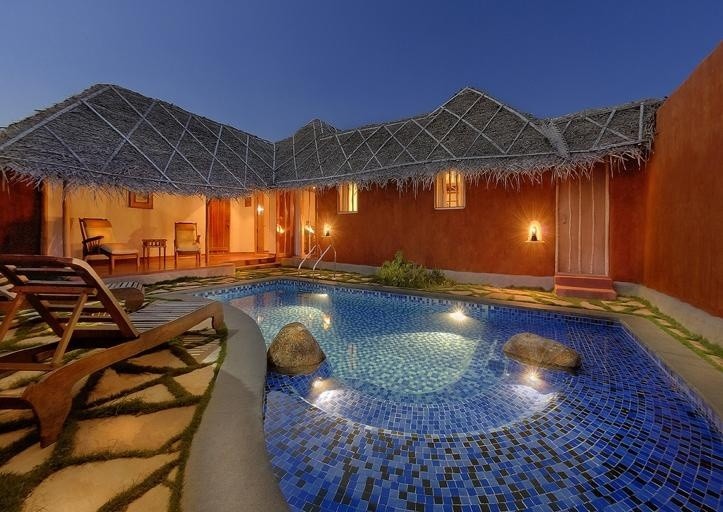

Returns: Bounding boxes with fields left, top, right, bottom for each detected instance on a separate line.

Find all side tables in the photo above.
left=142, top=238, right=168, bottom=268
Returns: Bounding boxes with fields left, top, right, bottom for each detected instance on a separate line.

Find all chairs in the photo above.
left=0, top=252, right=229, bottom=449
left=173, top=222, right=202, bottom=268
left=79, top=217, right=140, bottom=276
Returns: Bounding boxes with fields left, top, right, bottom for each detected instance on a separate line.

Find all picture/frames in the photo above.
left=128, top=189, right=153, bottom=208
